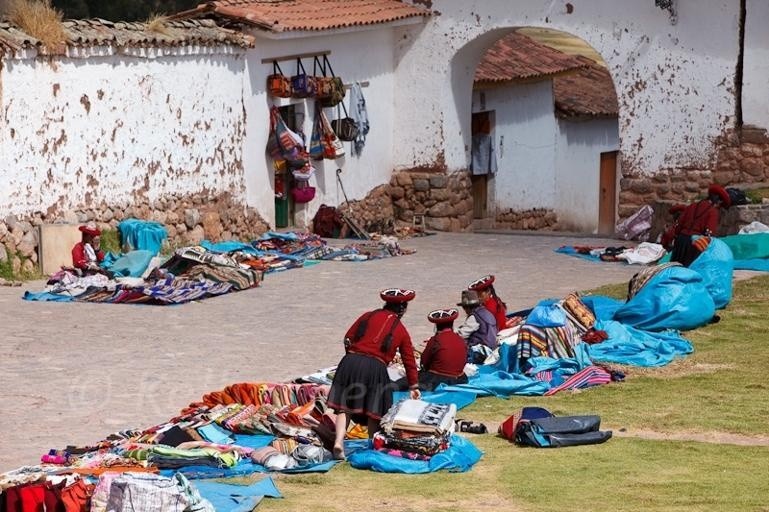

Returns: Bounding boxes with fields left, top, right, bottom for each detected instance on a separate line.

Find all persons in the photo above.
left=455, top=291, right=499, bottom=363
left=468, top=275, right=506, bottom=330
left=71, top=223, right=103, bottom=275
left=670, top=184, right=730, bottom=268
left=327, top=288, right=421, bottom=461
left=420, top=308, right=468, bottom=390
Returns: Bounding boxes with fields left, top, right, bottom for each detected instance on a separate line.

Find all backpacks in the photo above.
left=312, top=205, right=342, bottom=238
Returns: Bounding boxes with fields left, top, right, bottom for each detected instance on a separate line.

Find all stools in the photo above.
left=413, top=213, right=426, bottom=229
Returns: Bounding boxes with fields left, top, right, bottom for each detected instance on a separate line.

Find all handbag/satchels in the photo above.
left=292, top=181, right=317, bottom=204
left=265, top=106, right=347, bottom=177
left=318, top=55, right=346, bottom=105
left=330, top=99, right=358, bottom=140
left=264, top=61, right=291, bottom=98
left=306, top=58, right=335, bottom=98
left=288, top=60, right=318, bottom=98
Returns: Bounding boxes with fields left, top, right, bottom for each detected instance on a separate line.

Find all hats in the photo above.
left=457, top=289, right=480, bottom=307
left=78, top=224, right=101, bottom=237
left=710, top=182, right=732, bottom=208
left=468, top=274, right=496, bottom=293
left=378, top=287, right=416, bottom=303
left=426, top=306, right=458, bottom=323
left=468, top=296, right=471, bottom=300
left=670, top=205, right=686, bottom=213
